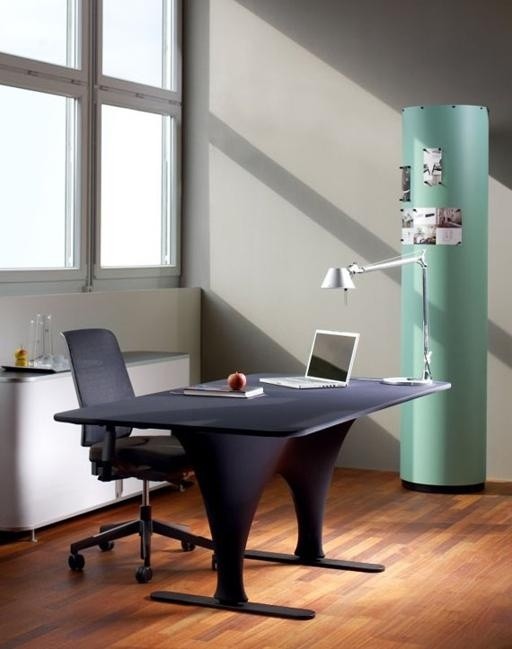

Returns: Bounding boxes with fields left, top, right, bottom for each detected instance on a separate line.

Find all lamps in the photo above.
left=320, top=246, right=433, bottom=387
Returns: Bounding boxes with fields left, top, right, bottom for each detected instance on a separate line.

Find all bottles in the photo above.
left=15, top=346, right=28, bottom=368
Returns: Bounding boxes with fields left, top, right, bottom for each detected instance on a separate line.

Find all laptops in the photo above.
left=259, top=328, right=360, bottom=391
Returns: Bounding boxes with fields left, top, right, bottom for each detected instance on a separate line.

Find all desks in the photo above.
left=0, top=349, right=190, bottom=541
left=53, top=372, right=452, bottom=618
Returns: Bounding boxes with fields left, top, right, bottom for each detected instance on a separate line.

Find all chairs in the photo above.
left=59, top=327, right=197, bottom=583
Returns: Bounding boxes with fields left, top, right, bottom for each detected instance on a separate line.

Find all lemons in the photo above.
left=15, top=348, right=27, bottom=361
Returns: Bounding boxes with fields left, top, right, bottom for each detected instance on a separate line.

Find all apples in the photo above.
left=227, top=373, right=246, bottom=389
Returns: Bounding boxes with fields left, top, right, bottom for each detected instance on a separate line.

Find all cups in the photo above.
left=30, top=313, right=53, bottom=367
